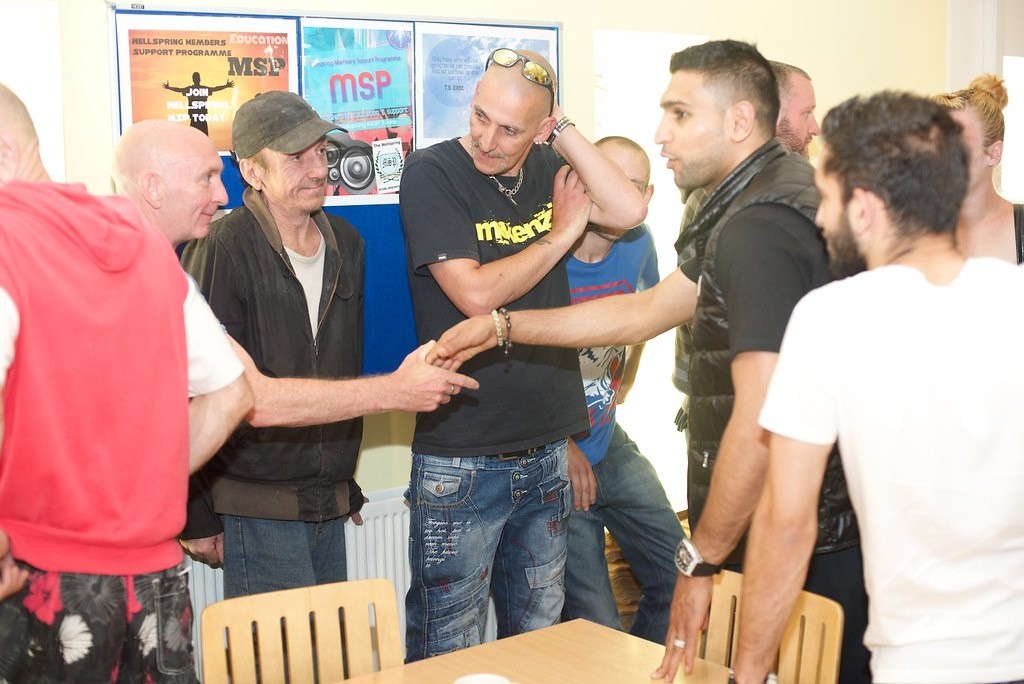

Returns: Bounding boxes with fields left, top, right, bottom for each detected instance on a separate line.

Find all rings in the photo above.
left=450, top=383, right=455, bottom=394
left=673, top=638, right=685, bottom=648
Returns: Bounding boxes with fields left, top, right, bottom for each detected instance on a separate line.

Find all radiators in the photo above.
left=183, top=484, right=497, bottom=684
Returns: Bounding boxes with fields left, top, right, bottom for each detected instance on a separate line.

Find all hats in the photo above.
left=232, top=90, right=348, bottom=159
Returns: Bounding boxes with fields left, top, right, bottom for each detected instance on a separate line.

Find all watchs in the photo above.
left=674, top=537, right=722, bottom=577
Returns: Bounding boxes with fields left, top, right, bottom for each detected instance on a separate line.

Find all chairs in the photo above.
left=201, top=577, right=404, bottom=683
left=695, top=569, right=845, bottom=684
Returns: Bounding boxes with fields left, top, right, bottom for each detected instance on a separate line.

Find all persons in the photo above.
left=174, top=90, right=366, bottom=597
left=563, top=39, right=1024, bottom=684
left=0, top=80, right=253, bottom=684
left=402, top=48, right=649, bottom=668
left=162, top=72, right=235, bottom=137
left=114, top=119, right=482, bottom=426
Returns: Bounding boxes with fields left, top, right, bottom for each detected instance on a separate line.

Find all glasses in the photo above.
left=486, top=48, right=554, bottom=117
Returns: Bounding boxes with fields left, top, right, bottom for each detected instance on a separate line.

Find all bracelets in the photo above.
left=491, top=309, right=503, bottom=346
left=500, top=306, right=512, bottom=353
left=547, top=115, right=574, bottom=144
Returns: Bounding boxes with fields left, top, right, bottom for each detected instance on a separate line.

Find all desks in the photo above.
left=340, top=618, right=730, bottom=684
